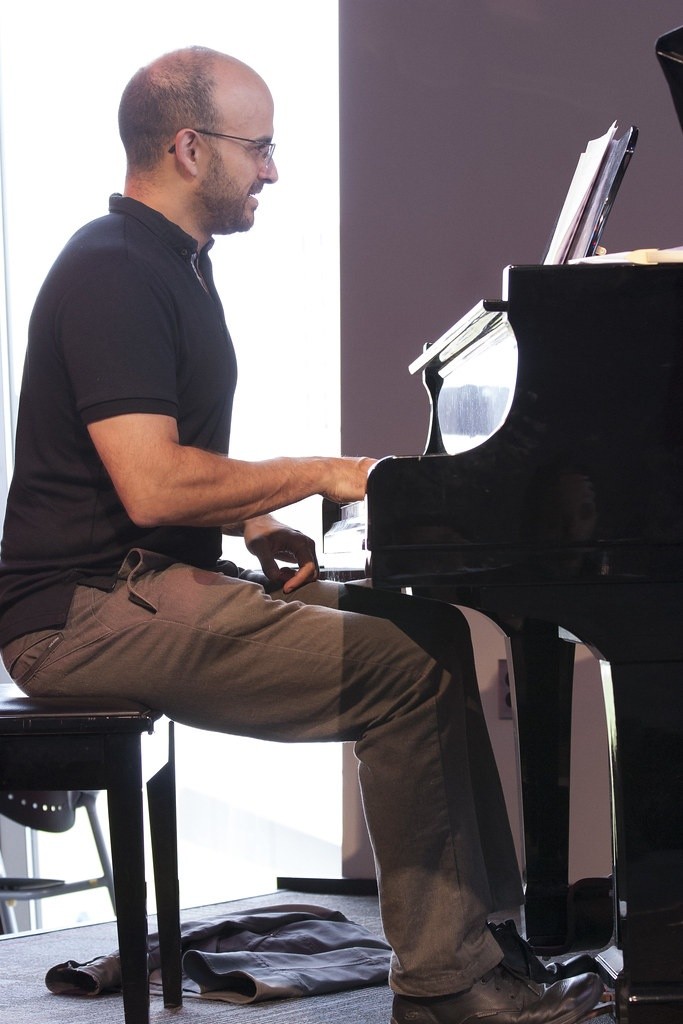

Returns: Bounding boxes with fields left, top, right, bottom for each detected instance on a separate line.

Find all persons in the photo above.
left=0, top=47, right=605, bottom=1024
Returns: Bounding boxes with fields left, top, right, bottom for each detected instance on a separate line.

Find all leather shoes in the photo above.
left=389, top=959, right=604, bottom=1024
left=489, top=919, right=600, bottom=985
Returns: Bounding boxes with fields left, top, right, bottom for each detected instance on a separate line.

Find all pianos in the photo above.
left=310, top=23, right=683, bottom=1023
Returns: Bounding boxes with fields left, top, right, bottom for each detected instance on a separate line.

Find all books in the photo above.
left=560, top=247, right=683, bottom=264
left=408, top=297, right=500, bottom=382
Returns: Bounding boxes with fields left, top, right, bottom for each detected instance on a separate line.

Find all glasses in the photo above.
left=168, top=129, right=276, bottom=165
